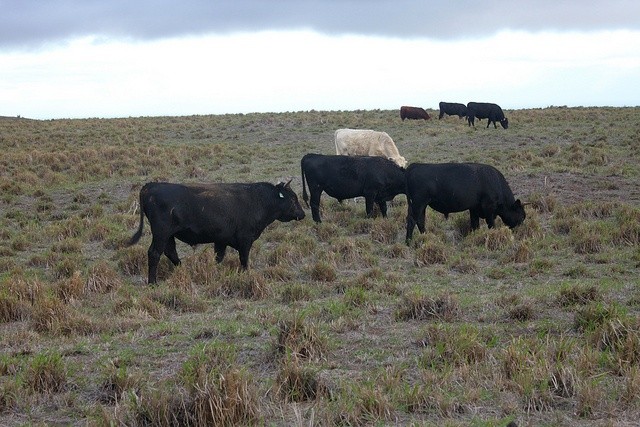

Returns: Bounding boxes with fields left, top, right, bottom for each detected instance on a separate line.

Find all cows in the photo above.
left=126, top=178, right=307, bottom=290
left=465, top=101, right=509, bottom=129
left=403, top=162, right=527, bottom=248
left=439, top=102, right=467, bottom=120
left=334, top=128, right=408, bottom=169
left=400, top=106, right=431, bottom=122
left=301, top=153, right=407, bottom=223
